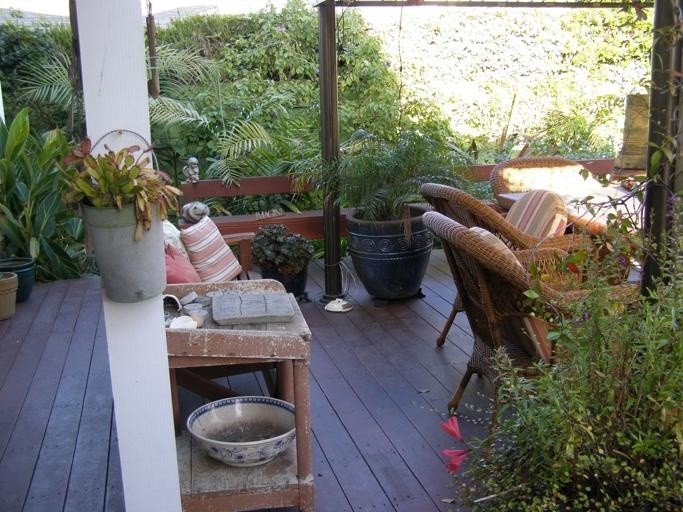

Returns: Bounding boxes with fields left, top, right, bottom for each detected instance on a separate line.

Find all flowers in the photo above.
left=440, top=299, right=682, bottom=512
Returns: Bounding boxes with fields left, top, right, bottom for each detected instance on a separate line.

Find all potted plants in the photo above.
left=55, top=137, right=184, bottom=303
left=249, top=225, right=314, bottom=303
left=287, top=129, right=473, bottom=308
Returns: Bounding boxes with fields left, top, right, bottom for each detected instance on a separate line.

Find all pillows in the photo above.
left=614, top=95, right=648, bottom=171
left=163, top=215, right=243, bottom=283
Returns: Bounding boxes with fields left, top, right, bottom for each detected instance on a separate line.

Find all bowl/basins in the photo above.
left=186, top=395, right=295, bottom=468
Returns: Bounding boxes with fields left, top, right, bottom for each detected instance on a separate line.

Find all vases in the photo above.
left=0, top=272, right=19, bottom=321
left=0, top=258, right=36, bottom=303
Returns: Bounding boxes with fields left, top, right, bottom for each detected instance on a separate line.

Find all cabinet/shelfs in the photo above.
left=163, top=279, right=315, bottom=512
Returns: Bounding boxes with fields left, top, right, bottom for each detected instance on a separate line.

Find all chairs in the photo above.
left=421, top=209, right=642, bottom=463
left=421, top=181, right=600, bottom=348
left=490, top=159, right=603, bottom=204
